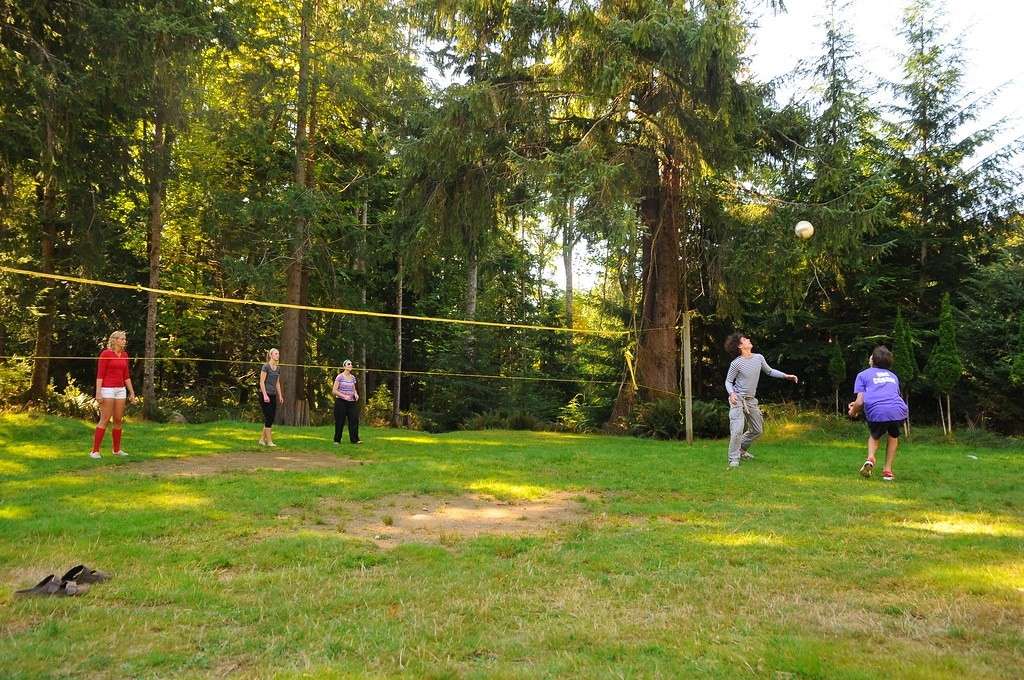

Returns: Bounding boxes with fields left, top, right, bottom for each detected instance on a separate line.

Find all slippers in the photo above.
left=62, top=563, right=114, bottom=587
left=12, top=573, right=91, bottom=599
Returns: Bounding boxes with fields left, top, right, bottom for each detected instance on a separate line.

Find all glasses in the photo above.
left=346, top=362, right=354, bottom=366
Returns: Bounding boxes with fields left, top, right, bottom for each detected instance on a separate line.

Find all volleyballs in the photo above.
left=795, top=220, right=815, bottom=238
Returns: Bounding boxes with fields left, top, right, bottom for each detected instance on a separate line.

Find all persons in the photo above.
left=332, top=359, right=362, bottom=446
left=89, top=331, right=136, bottom=459
left=258, top=348, right=284, bottom=446
left=725, top=332, right=798, bottom=467
left=848, top=345, right=909, bottom=480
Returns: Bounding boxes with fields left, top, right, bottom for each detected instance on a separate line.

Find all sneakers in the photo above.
left=90, top=450, right=101, bottom=458
left=113, top=449, right=129, bottom=456
left=729, top=461, right=739, bottom=468
left=859, top=457, right=876, bottom=478
left=882, top=469, right=895, bottom=481
left=741, top=452, right=755, bottom=462
